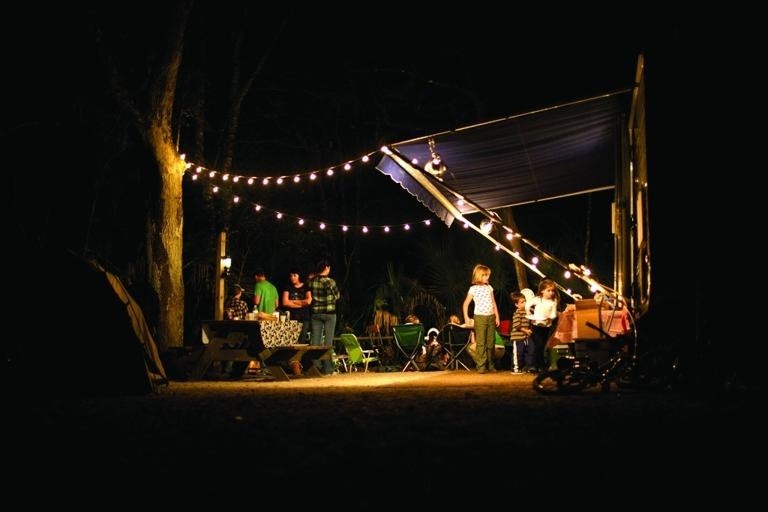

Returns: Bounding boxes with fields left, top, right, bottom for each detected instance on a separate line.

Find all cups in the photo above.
left=272, top=311, right=286, bottom=323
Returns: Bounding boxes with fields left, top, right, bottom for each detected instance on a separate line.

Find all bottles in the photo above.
left=252, top=304, right=259, bottom=321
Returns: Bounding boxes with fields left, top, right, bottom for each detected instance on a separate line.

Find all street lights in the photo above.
left=215, top=255, right=234, bottom=321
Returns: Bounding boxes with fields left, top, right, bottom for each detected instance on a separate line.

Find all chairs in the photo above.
left=306, top=332, right=348, bottom=377
left=388, top=325, right=426, bottom=372
left=340, top=333, right=385, bottom=374
left=436, top=323, right=472, bottom=372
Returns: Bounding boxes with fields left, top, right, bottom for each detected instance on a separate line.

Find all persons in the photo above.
left=524, top=279, right=561, bottom=374
left=508, top=292, right=533, bottom=375
left=404, top=315, right=420, bottom=326
left=461, top=315, right=506, bottom=372
left=461, top=264, right=500, bottom=373
left=223, top=260, right=341, bottom=377
left=443, top=315, right=460, bottom=365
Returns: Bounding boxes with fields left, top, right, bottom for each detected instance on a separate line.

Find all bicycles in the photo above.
left=532, top=347, right=659, bottom=393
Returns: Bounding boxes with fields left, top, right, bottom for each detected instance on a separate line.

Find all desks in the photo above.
left=164, top=345, right=337, bottom=383
left=333, top=336, right=455, bottom=370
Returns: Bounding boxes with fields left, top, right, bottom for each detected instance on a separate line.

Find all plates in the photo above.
left=524, top=314, right=547, bottom=321
